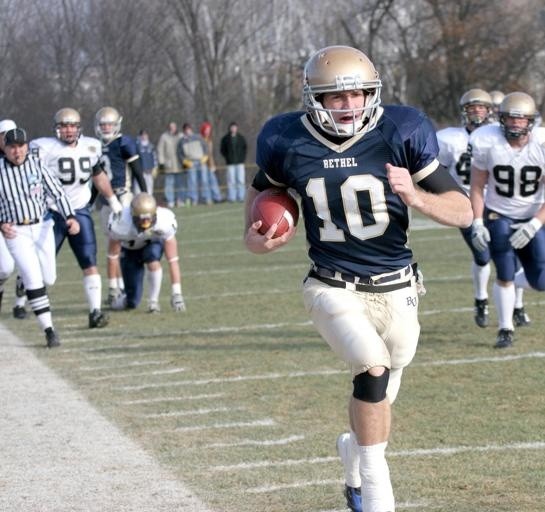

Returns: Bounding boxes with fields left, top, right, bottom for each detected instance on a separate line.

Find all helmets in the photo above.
left=303, top=46, right=382, bottom=138
left=132, top=193, right=155, bottom=230
left=0, top=107, right=121, bottom=145
left=459, top=88, right=538, bottom=140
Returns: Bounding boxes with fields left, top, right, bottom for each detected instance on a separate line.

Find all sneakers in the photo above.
left=2, top=288, right=189, bottom=347
left=474, top=298, right=530, bottom=350
left=166, top=194, right=245, bottom=209
left=336, top=433, right=361, bottom=505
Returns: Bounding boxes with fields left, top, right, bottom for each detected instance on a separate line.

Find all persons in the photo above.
left=242, top=45, right=474, bottom=512
left=1, top=106, right=186, bottom=346
left=221, top=122, right=248, bottom=203
left=436, top=88, right=545, bottom=349
left=134, top=120, right=223, bottom=208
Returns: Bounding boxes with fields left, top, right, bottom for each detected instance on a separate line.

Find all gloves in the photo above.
left=471, top=219, right=491, bottom=252
left=509, top=219, right=542, bottom=249
left=183, top=159, right=191, bottom=166
left=200, top=155, right=210, bottom=162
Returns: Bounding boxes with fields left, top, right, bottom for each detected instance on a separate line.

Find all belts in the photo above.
left=17, top=218, right=43, bottom=226
left=303, top=269, right=421, bottom=292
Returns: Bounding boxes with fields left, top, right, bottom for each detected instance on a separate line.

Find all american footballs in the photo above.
left=251, top=188, right=299, bottom=237
